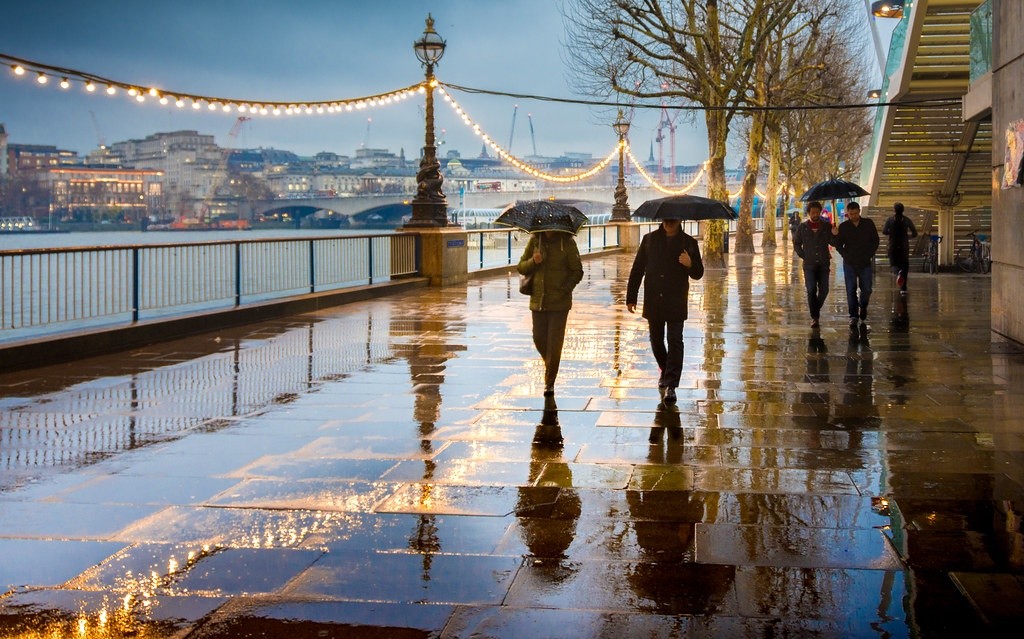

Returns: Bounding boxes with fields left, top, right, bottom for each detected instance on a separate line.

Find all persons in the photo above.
left=626, top=219, right=704, bottom=400
left=793, top=202, right=838, bottom=328
left=517, top=231, right=584, bottom=398
left=836, top=202, right=880, bottom=328
left=882, top=203, right=918, bottom=294
left=789, top=211, right=801, bottom=245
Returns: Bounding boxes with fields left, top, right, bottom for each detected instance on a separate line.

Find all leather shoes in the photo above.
left=658, top=374, right=676, bottom=399
left=544, top=384, right=555, bottom=396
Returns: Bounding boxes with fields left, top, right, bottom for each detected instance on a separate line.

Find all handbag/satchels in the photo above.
left=519, top=266, right=533, bottom=295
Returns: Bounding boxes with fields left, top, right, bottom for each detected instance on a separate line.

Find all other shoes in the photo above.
left=896, top=270, right=907, bottom=294
left=811, top=319, right=819, bottom=328
left=849, top=318, right=866, bottom=328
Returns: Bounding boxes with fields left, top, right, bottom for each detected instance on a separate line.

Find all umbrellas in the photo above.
left=799, top=174, right=871, bottom=227
left=630, top=194, right=740, bottom=252
left=494, top=201, right=590, bottom=254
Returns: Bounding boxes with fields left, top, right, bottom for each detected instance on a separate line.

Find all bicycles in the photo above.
left=921, top=232, right=943, bottom=274
left=956, top=230, right=991, bottom=274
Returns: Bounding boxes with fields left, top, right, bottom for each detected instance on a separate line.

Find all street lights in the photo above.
left=614, top=107, right=632, bottom=222
left=411, top=11, right=448, bottom=226
